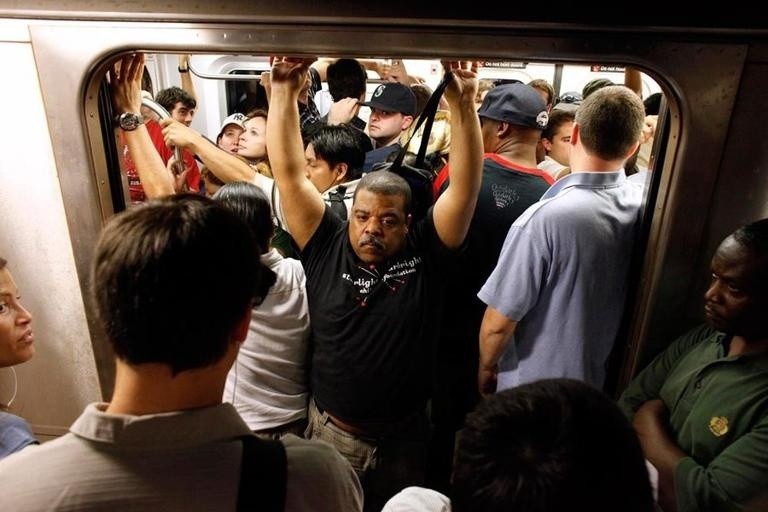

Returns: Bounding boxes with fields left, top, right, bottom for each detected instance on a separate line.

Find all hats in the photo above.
left=553, top=92, right=583, bottom=111
left=356, top=82, right=417, bottom=116
left=221, top=113, right=249, bottom=132
left=477, top=84, right=548, bottom=130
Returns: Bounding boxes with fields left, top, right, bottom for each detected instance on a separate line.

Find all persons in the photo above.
left=614, top=215, right=767, bottom=511
left=0, top=252, right=40, bottom=460
left=0, top=191, right=365, bottom=511
left=91, top=51, right=669, bottom=512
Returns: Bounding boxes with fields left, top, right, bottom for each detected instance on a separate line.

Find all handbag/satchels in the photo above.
left=373, top=161, right=433, bottom=219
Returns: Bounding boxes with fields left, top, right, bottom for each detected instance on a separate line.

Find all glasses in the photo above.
left=554, top=95, right=579, bottom=103
